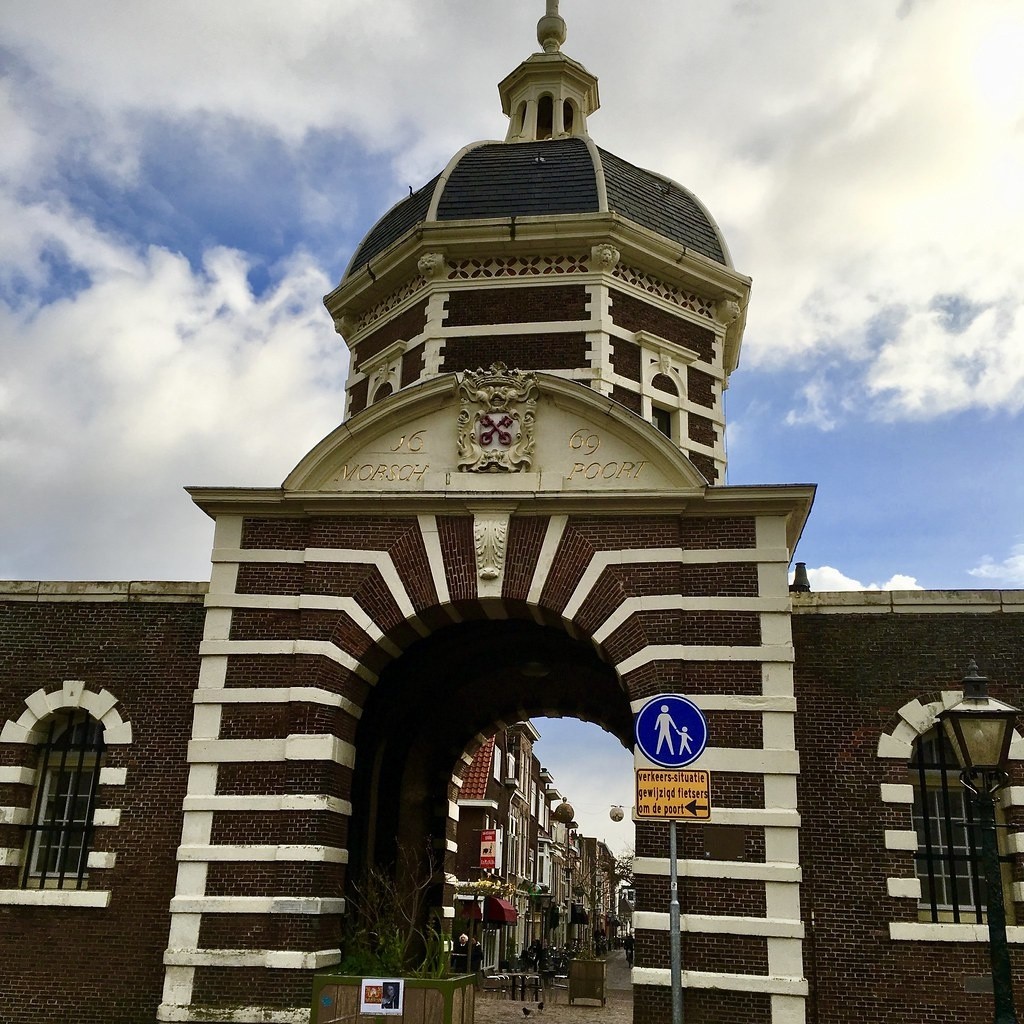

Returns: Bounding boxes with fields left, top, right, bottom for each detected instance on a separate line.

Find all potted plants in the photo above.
left=567, top=843, right=636, bottom=1008
left=309, top=835, right=477, bottom=1023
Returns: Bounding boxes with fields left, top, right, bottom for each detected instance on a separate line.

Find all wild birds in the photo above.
left=537, top=1001, right=544, bottom=1012
left=521, top=1007, right=532, bottom=1019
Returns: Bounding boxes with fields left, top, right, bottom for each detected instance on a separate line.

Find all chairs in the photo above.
left=482, top=945, right=570, bottom=1003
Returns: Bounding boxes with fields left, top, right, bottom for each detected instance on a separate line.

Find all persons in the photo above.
left=454, top=934, right=484, bottom=992
left=520, top=938, right=580, bottom=989
left=624, top=933, right=635, bottom=964
left=382, top=984, right=399, bottom=1009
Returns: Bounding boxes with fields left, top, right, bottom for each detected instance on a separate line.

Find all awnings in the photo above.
left=488, top=896, right=517, bottom=926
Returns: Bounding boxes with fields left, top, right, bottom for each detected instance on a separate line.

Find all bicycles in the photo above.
left=624, top=948, right=634, bottom=970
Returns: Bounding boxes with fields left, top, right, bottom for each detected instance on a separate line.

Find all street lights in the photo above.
left=934, top=658, right=1024, bottom=1024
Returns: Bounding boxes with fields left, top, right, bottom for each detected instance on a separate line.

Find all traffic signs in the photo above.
left=635, top=767, right=712, bottom=822
